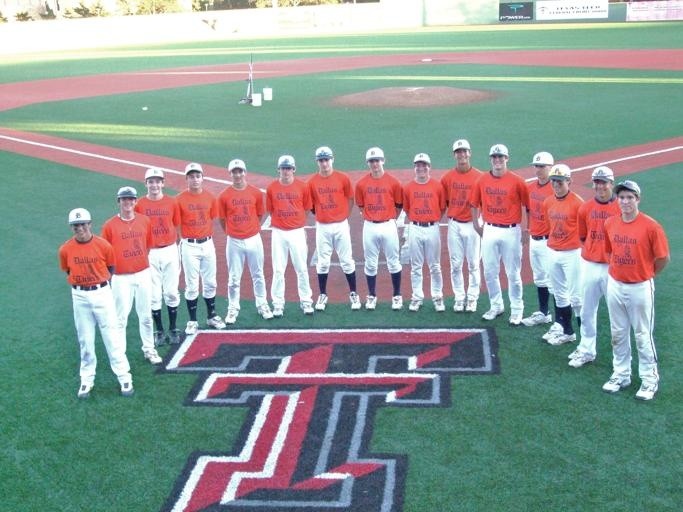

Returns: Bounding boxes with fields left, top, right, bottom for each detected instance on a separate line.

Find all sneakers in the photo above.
left=271, top=309, right=284, bottom=317
left=548, top=332, right=578, bottom=345
left=568, top=354, right=594, bottom=367
left=482, top=306, right=504, bottom=320
left=521, top=311, right=552, bottom=326
left=257, top=304, right=273, bottom=319
left=509, top=312, right=522, bottom=324
left=568, top=345, right=581, bottom=359
left=433, top=297, right=444, bottom=311
left=143, top=352, right=162, bottom=365
left=169, top=329, right=181, bottom=344
left=300, top=302, right=314, bottom=314
left=602, top=376, right=631, bottom=393
left=154, top=331, right=166, bottom=346
left=467, top=302, right=478, bottom=311
left=315, top=294, right=328, bottom=310
left=77, top=383, right=93, bottom=397
left=185, top=320, right=198, bottom=335
left=409, top=299, right=423, bottom=310
left=542, top=321, right=564, bottom=341
left=206, top=315, right=226, bottom=330
left=453, top=300, right=464, bottom=312
left=348, top=292, right=362, bottom=310
left=365, top=295, right=377, bottom=309
left=225, top=310, right=239, bottom=324
left=635, top=382, right=658, bottom=400
left=121, top=380, right=134, bottom=395
left=391, top=296, right=402, bottom=310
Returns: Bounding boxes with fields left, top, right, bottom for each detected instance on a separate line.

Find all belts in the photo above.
left=414, top=220, right=436, bottom=227
left=188, top=237, right=213, bottom=243
left=369, top=220, right=390, bottom=223
left=449, top=216, right=474, bottom=224
left=487, top=221, right=517, bottom=229
left=70, top=281, right=116, bottom=293
left=531, top=235, right=551, bottom=241
left=612, top=281, right=652, bottom=287
left=157, top=245, right=169, bottom=249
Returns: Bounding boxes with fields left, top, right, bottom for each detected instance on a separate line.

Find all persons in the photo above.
left=218, top=159, right=274, bottom=324
left=521, top=151, right=563, bottom=340
left=441, top=139, right=485, bottom=312
left=175, top=163, right=226, bottom=335
left=471, top=144, right=531, bottom=325
left=101, top=186, right=163, bottom=365
left=603, top=180, right=670, bottom=400
left=568, top=167, right=622, bottom=368
left=306, top=147, right=362, bottom=309
left=59, top=208, right=134, bottom=399
left=403, top=153, right=446, bottom=311
left=267, top=155, right=315, bottom=316
left=134, top=168, right=181, bottom=345
left=542, top=164, right=586, bottom=359
left=356, top=147, right=403, bottom=310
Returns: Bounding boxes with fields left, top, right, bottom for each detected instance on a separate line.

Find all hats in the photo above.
left=490, top=144, right=509, bottom=156
left=453, top=139, right=470, bottom=150
left=145, top=168, right=164, bottom=178
left=591, top=166, right=615, bottom=182
left=528, top=151, right=554, bottom=166
left=277, top=155, right=296, bottom=168
left=117, top=186, right=137, bottom=199
left=365, top=147, right=384, bottom=161
left=228, top=159, right=246, bottom=171
left=548, top=164, right=571, bottom=180
left=316, top=147, right=333, bottom=160
left=185, top=162, right=203, bottom=173
left=68, top=207, right=91, bottom=225
left=414, top=153, right=431, bottom=164
left=612, top=179, right=641, bottom=194
